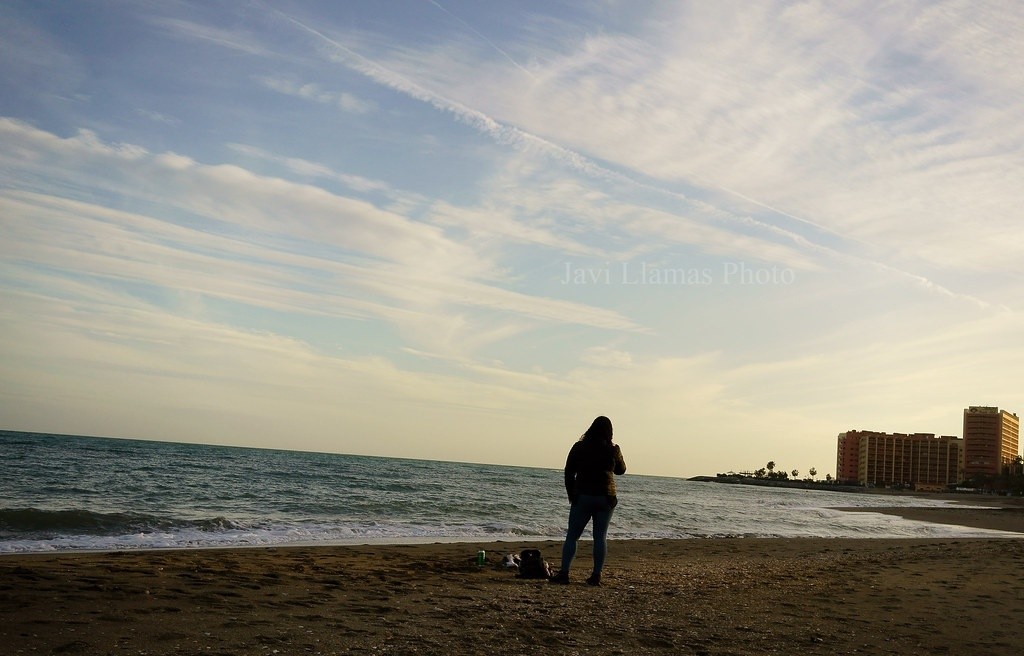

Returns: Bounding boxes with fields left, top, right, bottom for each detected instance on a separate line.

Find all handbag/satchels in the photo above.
left=519, top=549, right=550, bottom=578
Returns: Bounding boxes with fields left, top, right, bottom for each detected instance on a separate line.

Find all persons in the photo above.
left=548, top=416, right=627, bottom=585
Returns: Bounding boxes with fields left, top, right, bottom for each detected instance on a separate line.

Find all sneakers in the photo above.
left=549, top=570, right=570, bottom=584
left=586, top=572, right=602, bottom=585
